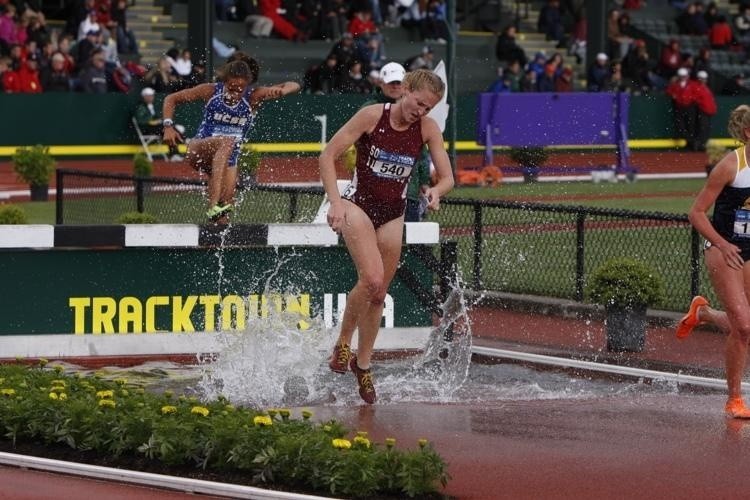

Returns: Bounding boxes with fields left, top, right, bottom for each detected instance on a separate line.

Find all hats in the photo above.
left=380, top=61, right=406, bottom=84
left=141, top=88, right=154, bottom=96
left=697, top=71, right=708, bottom=79
left=597, top=53, right=607, bottom=59
left=678, top=68, right=690, bottom=77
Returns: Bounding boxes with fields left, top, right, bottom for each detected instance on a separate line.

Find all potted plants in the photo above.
left=507, top=142, right=549, bottom=184
left=10, top=143, right=59, bottom=202
left=581, top=258, right=664, bottom=352
left=234, top=147, right=264, bottom=190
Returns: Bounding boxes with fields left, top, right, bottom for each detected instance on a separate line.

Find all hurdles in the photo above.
left=0, top=222, right=457, bottom=358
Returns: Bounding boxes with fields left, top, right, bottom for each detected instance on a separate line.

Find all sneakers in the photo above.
left=676, top=295, right=710, bottom=339
left=328, top=339, right=351, bottom=374
left=206, top=203, right=234, bottom=225
left=350, top=357, right=376, bottom=405
left=725, top=396, right=750, bottom=418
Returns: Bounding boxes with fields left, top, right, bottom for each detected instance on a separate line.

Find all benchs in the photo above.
left=35, top=0, right=588, bottom=95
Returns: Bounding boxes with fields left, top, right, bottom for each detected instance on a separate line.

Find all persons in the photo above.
left=217, top=2, right=450, bottom=45
left=161, top=51, right=301, bottom=222
left=676, top=105, right=750, bottom=420
left=317, top=70, right=455, bottom=404
left=667, top=69, right=718, bottom=153
left=301, top=33, right=434, bottom=95
left=538, top=1, right=748, bottom=93
left=145, top=46, right=206, bottom=95
left=1, top=1, right=139, bottom=93
left=485, top=24, right=575, bottom=93
left=359, top=60, right=432, bottom=221
left=135, top=88, right=191, bottom=145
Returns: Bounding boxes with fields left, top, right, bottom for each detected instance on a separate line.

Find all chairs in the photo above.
left=616, top=1, right=750, bottom=91
